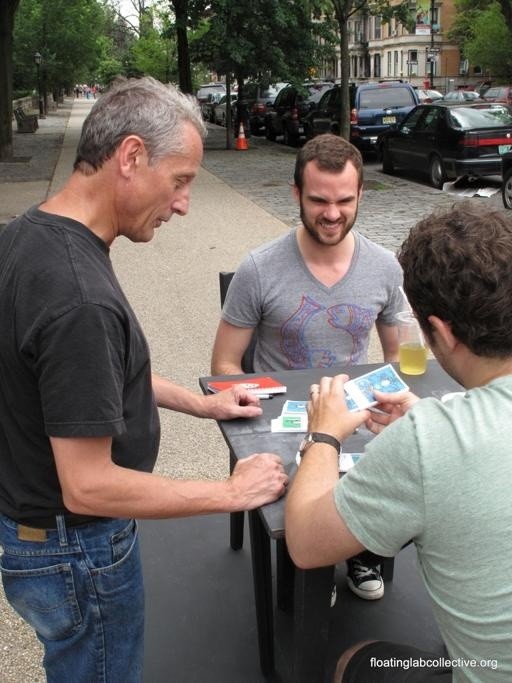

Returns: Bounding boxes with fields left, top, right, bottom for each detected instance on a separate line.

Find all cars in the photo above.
left=374, top=83, right=512, bottom=189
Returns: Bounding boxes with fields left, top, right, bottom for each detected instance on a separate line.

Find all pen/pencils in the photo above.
left=256, top=394, right=274, bottom=400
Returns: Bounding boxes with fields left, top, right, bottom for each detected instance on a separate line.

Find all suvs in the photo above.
left=196, top=78, right=422, bottom=162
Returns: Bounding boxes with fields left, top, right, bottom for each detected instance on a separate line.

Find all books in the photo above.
left=206, top=373, right=287, bottom=400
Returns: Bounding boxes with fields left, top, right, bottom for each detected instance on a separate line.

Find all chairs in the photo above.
left=219, top=270, right=260, bottom=554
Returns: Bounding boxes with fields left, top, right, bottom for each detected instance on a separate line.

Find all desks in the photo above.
left=196, top=357, right=464, bottom=683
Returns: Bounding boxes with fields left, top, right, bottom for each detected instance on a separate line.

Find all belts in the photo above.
left=18, top=511, right=106, bottom=530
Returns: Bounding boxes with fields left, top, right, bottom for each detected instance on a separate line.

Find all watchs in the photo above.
left=299, top=431, right=341, bottom=457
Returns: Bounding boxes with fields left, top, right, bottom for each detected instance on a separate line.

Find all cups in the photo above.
left=395, top=310, right=428, bottom=376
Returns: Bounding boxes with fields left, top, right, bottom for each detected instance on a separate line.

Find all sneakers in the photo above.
left=346, top=560, right=386, bottom=602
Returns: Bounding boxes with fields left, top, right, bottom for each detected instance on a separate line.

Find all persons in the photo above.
left=281, top=197, right=510, bottom=682
left=1, top=74, right=288, bottom=683
left=208, top=132, right=416, bottom=603
left=73, top=83, right=97, bottom=98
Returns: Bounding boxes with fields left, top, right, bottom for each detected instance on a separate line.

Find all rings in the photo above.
left=310, top=390, right=319, bottom=396
